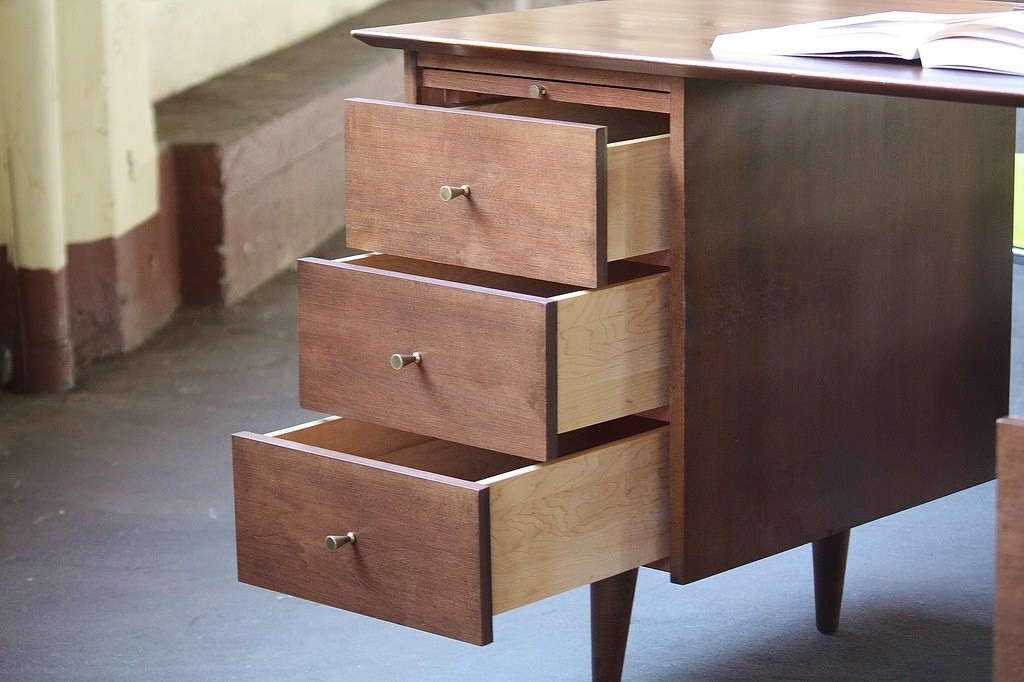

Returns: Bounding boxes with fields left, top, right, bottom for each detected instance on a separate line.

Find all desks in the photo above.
left=350, top=0, right=1024, bottom=682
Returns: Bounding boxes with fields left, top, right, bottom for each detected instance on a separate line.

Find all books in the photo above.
left=710, top=10, right=1024, bottom=75
